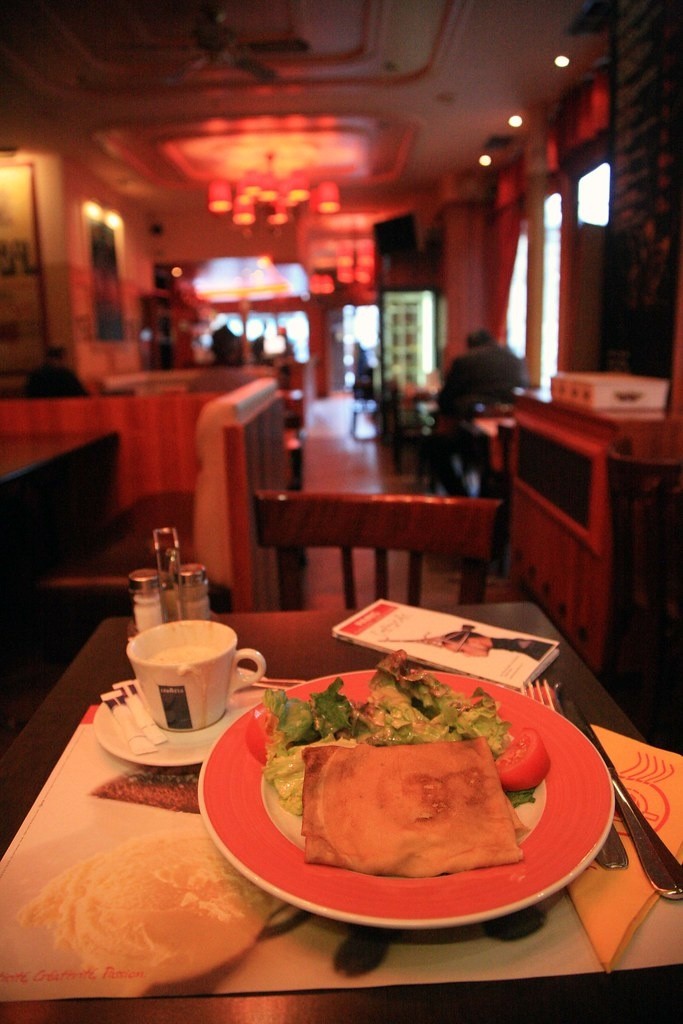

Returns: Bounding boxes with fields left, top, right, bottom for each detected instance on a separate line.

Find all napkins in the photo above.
left=564, top=719, right=683, bottom=971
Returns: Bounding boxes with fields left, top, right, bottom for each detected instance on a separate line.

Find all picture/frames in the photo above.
left=73, top=189, right=134, bottom=353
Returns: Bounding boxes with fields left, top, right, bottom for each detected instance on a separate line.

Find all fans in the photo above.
left=117, top=0, right=313, bottom=95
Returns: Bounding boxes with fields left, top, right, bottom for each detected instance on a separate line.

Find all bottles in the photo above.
left=127, top=569, right=167, bottom=634
left=153, top=528, right=181, bottom=622
left=176, top=563, right=211, bottom=620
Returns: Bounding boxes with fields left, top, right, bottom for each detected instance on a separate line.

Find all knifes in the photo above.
left=553, top=681, right=683, bottom=900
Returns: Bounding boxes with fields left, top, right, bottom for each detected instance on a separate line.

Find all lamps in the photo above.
left=207, top=141, right=341, bottom=223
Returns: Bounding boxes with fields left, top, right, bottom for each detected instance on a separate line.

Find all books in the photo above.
left=333, top=597, right=561, bottom=689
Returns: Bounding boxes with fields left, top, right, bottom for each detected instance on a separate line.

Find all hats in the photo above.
left=210, top=325, right=241, bottom=348
left=465, top=331, right=493, bottom=347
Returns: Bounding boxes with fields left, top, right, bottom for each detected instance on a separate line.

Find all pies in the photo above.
left=300, top=737, right=526, bottom=878
left=13, top=829, right=287, bottom=996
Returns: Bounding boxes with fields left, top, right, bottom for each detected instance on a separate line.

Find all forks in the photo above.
left=521, top=679, right=629, bottom=871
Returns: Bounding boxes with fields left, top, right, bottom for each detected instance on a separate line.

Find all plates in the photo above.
left=196, top=669, right=616, bottom=930
left=94, top=678, right=278, bottom=767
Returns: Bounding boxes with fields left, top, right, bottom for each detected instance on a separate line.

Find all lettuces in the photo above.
left=263, top=647, right=538, bottom=816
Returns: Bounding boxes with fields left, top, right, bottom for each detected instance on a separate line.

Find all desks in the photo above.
left=0, top=428, right=120, bottom=551
left=511, top=383, right=683, bottom=680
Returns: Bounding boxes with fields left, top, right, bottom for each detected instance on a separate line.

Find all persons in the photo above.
left=351, top=344, right=369, bottom=378
left=432, top=326, right=521, bottom=497
left=441, top=624, right=553, bottom=661
left=210, top=319, right=293, bottom=368
left=25, top=342, right=88, bottom=398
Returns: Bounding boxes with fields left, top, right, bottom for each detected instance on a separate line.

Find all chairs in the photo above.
left=388, top=378, right=438, bottom=476
left=252, top=488, right=500, bottom=614
left=583, top=451, right=683, bottom=756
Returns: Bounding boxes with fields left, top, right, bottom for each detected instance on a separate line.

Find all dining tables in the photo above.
left=0, top=600, right=683, bottom=1024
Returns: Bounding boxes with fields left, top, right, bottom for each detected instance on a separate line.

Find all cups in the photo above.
left=125, top=618, right=267, bottom=733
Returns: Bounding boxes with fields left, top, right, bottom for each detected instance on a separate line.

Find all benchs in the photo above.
left=42, top=378, right=288, bottom=669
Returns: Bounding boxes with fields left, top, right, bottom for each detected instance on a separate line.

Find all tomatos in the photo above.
left=495, top=727, right=551, bottom=791
